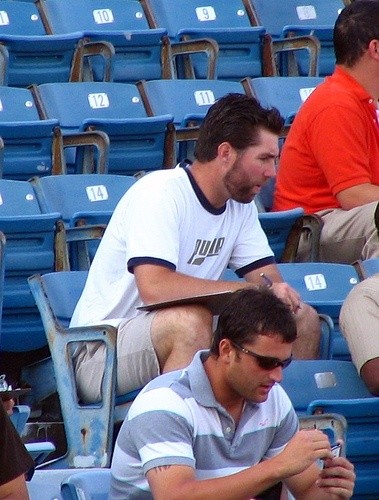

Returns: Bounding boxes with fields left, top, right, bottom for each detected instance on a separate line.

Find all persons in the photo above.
left=109, top=285, right=358, bottom=500
left=271, top=0, right=379, bottom=265
left=339, top=205, right=379, bottom=397
left=0, top=374, right=35, bottom=500
left=70, top=92, right=319, bottom=407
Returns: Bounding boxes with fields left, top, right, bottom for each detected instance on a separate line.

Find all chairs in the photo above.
left=0, top=0, right=379, bottom=500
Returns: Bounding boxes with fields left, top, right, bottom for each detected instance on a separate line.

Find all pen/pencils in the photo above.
left=261, top=273, right=301, bottom=312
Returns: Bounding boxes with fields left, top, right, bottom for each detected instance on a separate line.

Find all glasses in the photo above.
left=231, top=341, right=293, bottom=370
